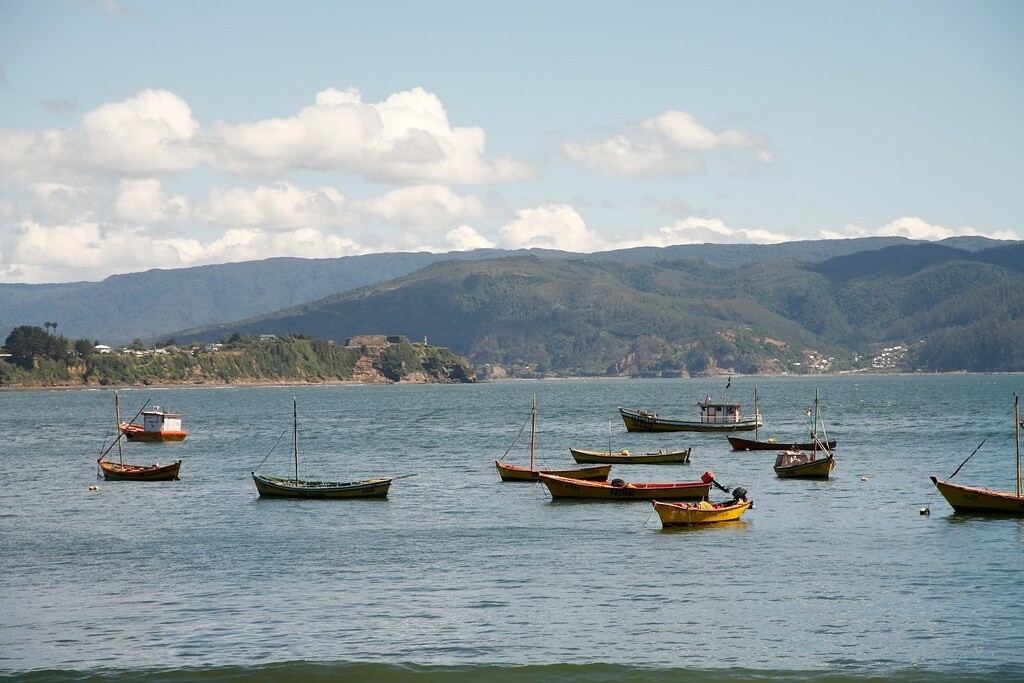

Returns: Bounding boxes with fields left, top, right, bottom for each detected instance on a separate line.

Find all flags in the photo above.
left=726, top=378, right=730, bottom=389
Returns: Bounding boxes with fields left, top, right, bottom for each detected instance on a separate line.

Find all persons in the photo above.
left=782, top=452, right=788, bottom=465
left=810, top=454, right=814, bottom=461
left=651, top=413, right=657, bottom=422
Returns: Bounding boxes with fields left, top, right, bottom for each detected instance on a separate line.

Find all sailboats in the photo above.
left=773, top=386, right=835, bottom=481
left=495, top=392, right=614, bottom=485
left=928, top=394, right=1024, bottom=513
left=96, top=393, right=183, bottom=482
left=250, top=397, right=393, bottom=499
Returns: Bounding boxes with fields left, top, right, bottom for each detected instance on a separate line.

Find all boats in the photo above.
left=568, top=420, right=691, bottom=465
left=616, top=384, right=764, bottom=431
left=649, top=486, right=755, bottom=528
left=119, top=405, right=188, bottom=442
left=538, top=470, right=732, bottom=499
left=725, top=384, right=837, bottom=451
